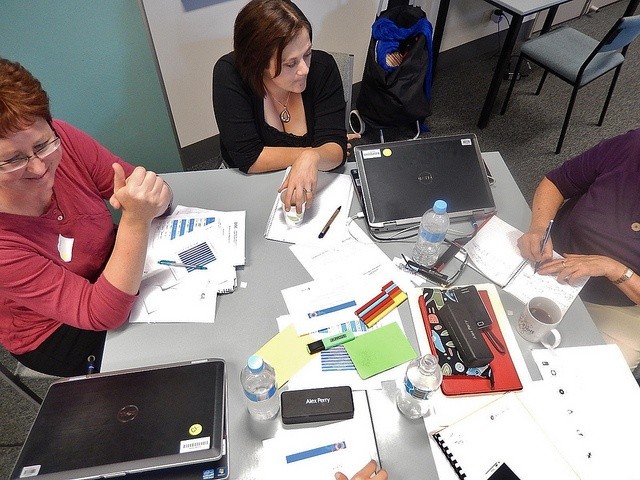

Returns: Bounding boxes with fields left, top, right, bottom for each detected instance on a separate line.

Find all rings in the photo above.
left=305, top=189, right=313, bottom=193
left=562, top=261, right=566, bottom=268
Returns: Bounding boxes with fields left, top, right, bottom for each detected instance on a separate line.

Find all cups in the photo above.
left=516, top=297, right=563, bottom=349
left=280, top=189, right=306, bottom=227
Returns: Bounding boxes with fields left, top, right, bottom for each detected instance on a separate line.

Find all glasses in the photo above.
left=402, top=237, right=469, bottom=287
left=0, top=119, right=60, bottom=174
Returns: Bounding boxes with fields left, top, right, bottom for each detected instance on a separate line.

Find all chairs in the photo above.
left=0, top=359, right=60, bottom=409
left=500, top=15, right=640, bottom=153
left=331, top=51, right=354, bottom=134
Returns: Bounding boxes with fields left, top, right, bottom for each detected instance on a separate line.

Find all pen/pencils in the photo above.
left=358, top=283, right=396, bottom=318
left=318, top=206, right=341, bottom=238
left=366, top=292, right=408, bottom=328
left=364, top=289, right=402, bottom=324
left=361, top=286, right=400, bottom=321
left=405, top=264, right=448, bottom=286
left=158, top=260, right=207, bottom=269
left=534, top=220, right=554, bottom=273
left=355, top=281, right=394, bottom=315
left=408, top=260, right=448, bottom=280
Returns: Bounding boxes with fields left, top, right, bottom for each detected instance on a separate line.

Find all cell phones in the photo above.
left=453, top=285, right=493, bottom=331
left=482, top=461, right=520, bottom=480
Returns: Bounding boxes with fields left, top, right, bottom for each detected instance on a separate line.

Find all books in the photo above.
left=261, top=165, right=355, bottom=245
left=251, top=390, right=389, bottom=480
left=430, top=380, right=598, bottom=480
left=138, top=212, right=247, bottom=272
left=384, top=51, right=409, bottom=67
left=454, top=213, right=593, bottom=325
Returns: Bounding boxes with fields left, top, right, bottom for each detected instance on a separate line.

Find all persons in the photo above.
left=517, top=127, right=640, bottom=373
left=211, top=1, right=348, bottom=215
left=0, top=57, right=172, bottom=379
left=334, top=461, right=391, bottom=480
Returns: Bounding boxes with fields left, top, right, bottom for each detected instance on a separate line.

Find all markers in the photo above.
left=306, top=331, right=355, bottom=354
left=431, top=239, right=464, bottom=272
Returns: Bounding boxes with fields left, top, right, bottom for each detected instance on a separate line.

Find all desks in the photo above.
left=99, top=152, right=640, bottom=480
left=430, top=0, right=639, bottom=130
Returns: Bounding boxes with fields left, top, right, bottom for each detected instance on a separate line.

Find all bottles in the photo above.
left=412, top=200, right=450, bottom=267
left=240, top=355, right=280, bottom=420
left=396, top=354, right=443, bottom=419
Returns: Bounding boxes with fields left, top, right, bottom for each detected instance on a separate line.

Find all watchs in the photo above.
left=613, top=267, right=634, bottom=286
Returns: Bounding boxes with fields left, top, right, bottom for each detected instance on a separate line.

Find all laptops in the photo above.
left=349, top=133, right=498, bottom=234
left=8, top=356, right=229, bottom=480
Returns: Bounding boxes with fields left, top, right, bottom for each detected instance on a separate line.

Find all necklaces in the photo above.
left=268, top=91, right=294, bottom=123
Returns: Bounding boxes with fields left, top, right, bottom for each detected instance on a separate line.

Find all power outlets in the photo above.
left=490, top=8, right=504, bottom=24
